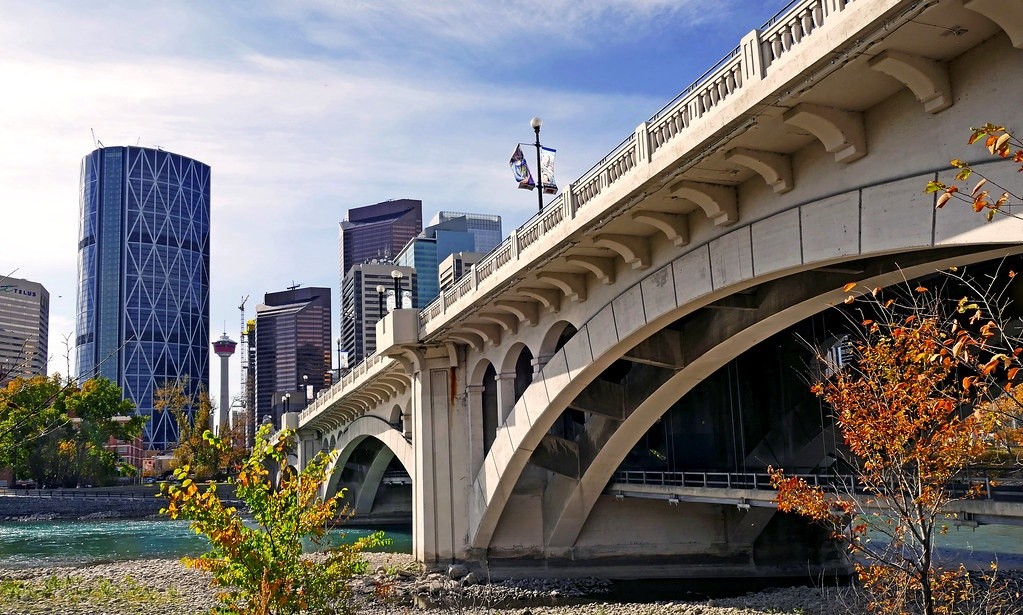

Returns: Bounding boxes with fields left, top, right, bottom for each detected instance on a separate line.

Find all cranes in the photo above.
left=287, top=281, right=302, bottom=291
left=238, top=294, right=250, bottom=450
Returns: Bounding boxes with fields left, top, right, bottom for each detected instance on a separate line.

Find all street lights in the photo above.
left=530, top=115, right=542, bottom=217
left=376, top=284, right=385, bottom=320
left=391, top=270, right=401, bottom=309
left=398, top=271, right=403, bottom=309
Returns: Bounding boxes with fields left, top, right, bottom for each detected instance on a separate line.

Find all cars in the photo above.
left=111, top=464, right=241, bottom=485
